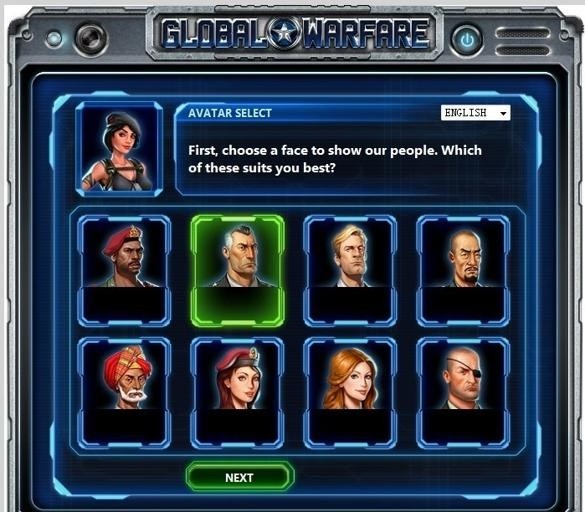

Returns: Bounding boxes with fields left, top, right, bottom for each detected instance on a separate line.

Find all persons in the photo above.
left=443, top=229, right=486, bottom=287
left=89, top=226, right=160, bottom=287
left=322, top=348, right=379, bottom=409
left=213, top=347, right=263, bottom=410
left=210, top=224, right=276, bottom=287
left=81, top=111, right=154, bottom=192
left=441, top=349, right=484, bottom=409
left=331, top=226, right=376, bottom=289
left=102, top=344, right=154, bottom=409
